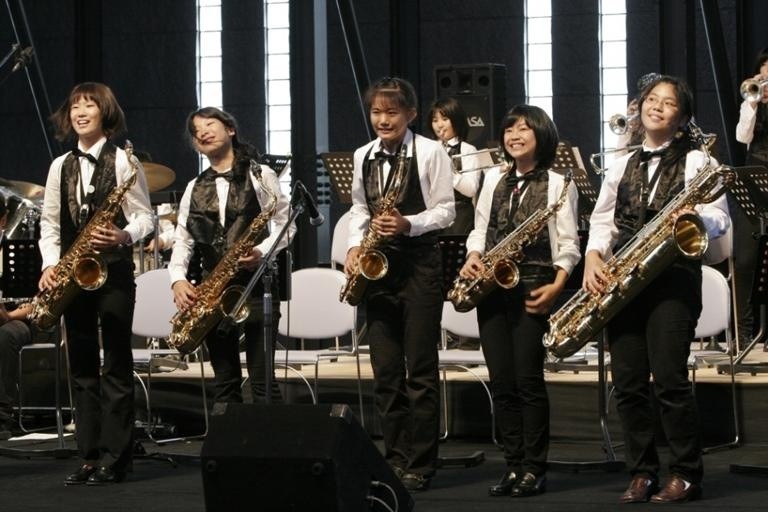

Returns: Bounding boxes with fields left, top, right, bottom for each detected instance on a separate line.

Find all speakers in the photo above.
left=433, top=62, right=507, bottom=150
left=200, top=402, right=414, bottom=511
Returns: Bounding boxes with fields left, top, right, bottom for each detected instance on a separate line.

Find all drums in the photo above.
left=154, top=202, right=179, bottom=249
left=133, top=248, right=164, bottom=279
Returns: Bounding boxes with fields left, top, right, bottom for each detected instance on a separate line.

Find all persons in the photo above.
left=166, top=108, right=298, bottom=406
left=3, top=49, right=768, bottom=438
left=343, top=76, right=457, bottom=493
left=460, top=103, right=582, bottom=497
left=40, top=80, right=155, bottom=487
left=585, top=74, right=729, bottom=505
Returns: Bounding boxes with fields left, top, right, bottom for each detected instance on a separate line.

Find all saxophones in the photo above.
left=166, top=160, right=277, bottom=354
left=448, top=168, right=572, bottom=312
left=27, top=141, right=138, bottom=331
left=340, top=144, right=409, bottom=305
left=542, top=135, right=737, bottom=357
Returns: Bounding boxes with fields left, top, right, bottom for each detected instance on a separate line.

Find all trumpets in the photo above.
left=741, top=77, right=767, bottom=100
left=609, top=112, right=640, bottom=133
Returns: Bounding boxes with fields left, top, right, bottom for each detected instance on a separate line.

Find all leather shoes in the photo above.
left=392, top=466, right=403, bottom=480
left=488, top=471, right=518, bottom=496
left=85, top=466, right=128, bottom=485
left=619, top=478, right=657, bottom=502
left=400, top=472, right=428, bottom=493
left=510, top=471, right=546, bottom=497
left=63, top=464, right=95, bottom=484
left=649, top=476, right=696, bottom=503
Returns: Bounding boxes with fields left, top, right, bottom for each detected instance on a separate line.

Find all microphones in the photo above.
left=298, top=180, right=324, bottom=226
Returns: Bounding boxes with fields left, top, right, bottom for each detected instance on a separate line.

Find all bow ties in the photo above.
left=504, top=169, right=538, bottom=187
left=205, top=169, right=234, bottom=182
left=72, top=147, right=97, bottom=163
left=374, top=151, right=396, bottom=166
left=639, top=150, right=664, bottom=162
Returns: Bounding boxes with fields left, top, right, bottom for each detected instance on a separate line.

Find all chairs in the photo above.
left=15, top=315, right=76, bottom=438
left=238, top=264, right=368, bottom=431
left=437, top=301, right=502, bottom=447
left=100, top=267, right=214, bottom=446
left=603, top=263, right=742, bottom=459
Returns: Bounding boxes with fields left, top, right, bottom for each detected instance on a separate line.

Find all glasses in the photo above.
left=643, top=93, right=679, bottom=110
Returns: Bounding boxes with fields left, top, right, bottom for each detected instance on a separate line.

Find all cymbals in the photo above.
left=140, top=162, right=177, bottom=193
left=0, top=180, right=45, bottom=208
